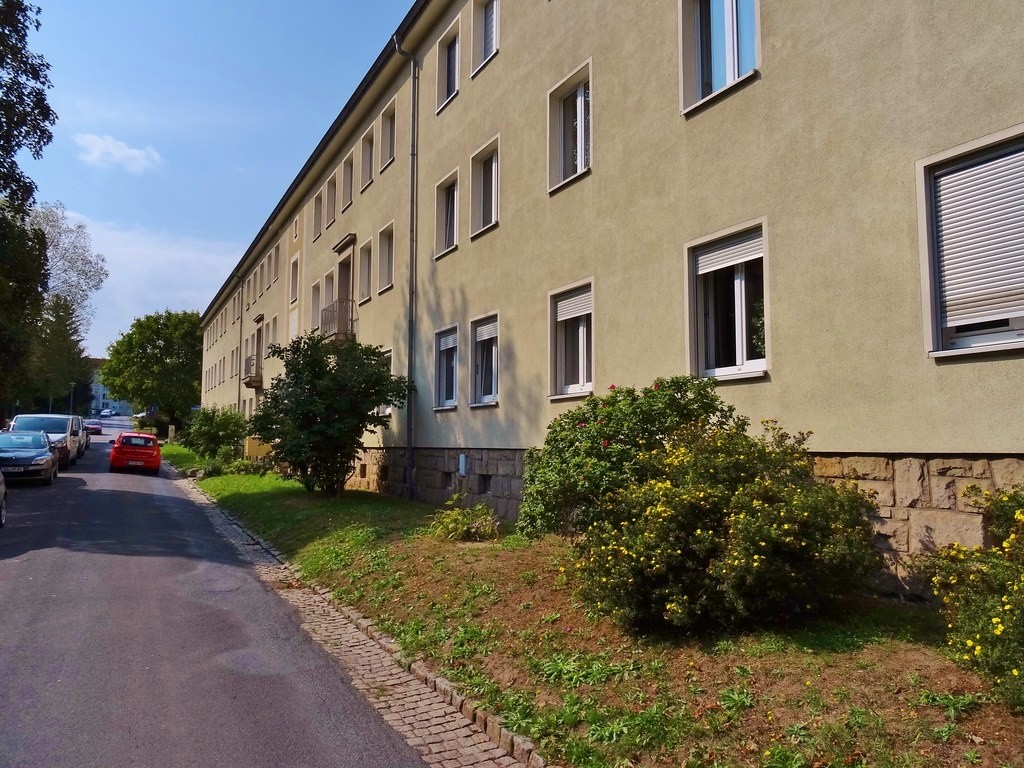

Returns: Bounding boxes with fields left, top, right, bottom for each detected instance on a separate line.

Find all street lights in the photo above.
left=69, top=382, right=76, bottom=414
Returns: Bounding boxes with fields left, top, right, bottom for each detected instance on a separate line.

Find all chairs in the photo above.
left=144, top=439, right=151, bottom=446
left=2, top=435, right=14, bottom=446
left=32, top=436, right=42, bottom=448
left=125, top=437, right=132, bottom=444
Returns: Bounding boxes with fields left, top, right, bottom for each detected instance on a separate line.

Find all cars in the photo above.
left=84, top=420, right=103, bottom=435
left=0, top=430, right=59, bottom=484
left=100, top=409, right=120, bottom=418
left=108, top=432, right=164, bottom=475
left=9, top=414, right=91, bottom=470
